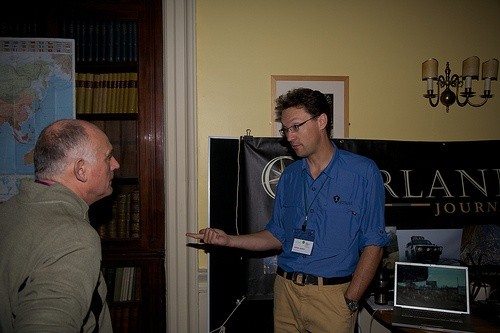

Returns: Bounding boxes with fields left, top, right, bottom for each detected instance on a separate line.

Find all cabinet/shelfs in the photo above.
left=0, top=0, right=167, bottom=333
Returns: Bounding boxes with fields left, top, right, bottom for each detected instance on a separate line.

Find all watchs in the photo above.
left=345, top=297, right=358, bottom=311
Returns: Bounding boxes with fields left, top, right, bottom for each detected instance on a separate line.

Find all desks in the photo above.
left=362, top=296, right=500, bottom=333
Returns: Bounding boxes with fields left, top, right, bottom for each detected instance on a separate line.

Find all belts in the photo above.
left=275, top=266, right=353, bottom=286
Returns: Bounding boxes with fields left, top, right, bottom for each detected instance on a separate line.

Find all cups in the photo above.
left=470, top=282, right=491, bottom=304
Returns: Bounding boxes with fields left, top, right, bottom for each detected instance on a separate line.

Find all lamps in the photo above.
left=422, top=56, right=499, bottom=113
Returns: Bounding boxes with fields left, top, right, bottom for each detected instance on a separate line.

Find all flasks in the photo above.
left=374, top=270, right=389, bottom=305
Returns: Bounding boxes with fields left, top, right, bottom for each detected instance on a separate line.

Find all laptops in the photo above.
left=392, top=260, right=475, bottom=333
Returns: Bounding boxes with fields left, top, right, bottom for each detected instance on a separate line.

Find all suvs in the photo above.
left=404, top=236, right=443, bottom=264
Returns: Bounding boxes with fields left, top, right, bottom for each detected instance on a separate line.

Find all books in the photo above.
left=24, top=17, right=141, bottom=303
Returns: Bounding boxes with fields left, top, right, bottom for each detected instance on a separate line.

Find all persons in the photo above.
left=0, top=119, right=119, bottom=333
left=186, top=87, right=387, bottom=333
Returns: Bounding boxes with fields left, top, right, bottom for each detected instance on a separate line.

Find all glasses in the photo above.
left=279, top=114, right=318, bottom=137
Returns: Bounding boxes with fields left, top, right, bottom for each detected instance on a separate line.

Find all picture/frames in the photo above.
left=271, top=75, right=349, bottom=139
